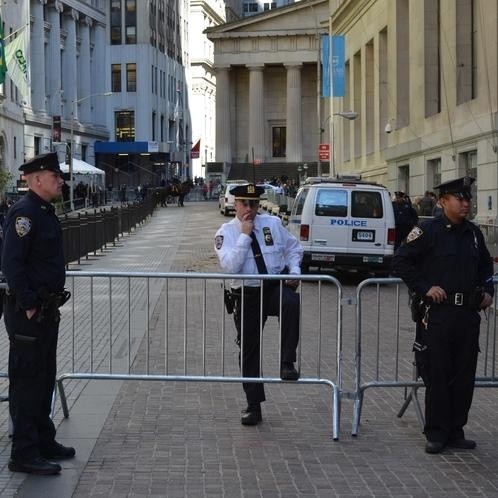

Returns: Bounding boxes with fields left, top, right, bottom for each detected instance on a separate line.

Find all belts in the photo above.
left=415, top=292, right=482, bottom=305
left=231, top=273, right=286, bottom=295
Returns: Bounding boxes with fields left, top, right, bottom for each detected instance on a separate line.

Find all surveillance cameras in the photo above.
left=385, top=124, right=392, bottom=133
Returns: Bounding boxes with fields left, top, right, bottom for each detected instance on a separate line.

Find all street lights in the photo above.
left=52, top=91, right=115, bottom=210
left=315, top=109, right=358, bottom=174
left=296, top=164, right=302, bottom=181
left=302, top=162, right=309, bottom=179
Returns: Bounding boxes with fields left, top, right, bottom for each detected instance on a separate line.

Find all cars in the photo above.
left=255, top=183, right=282, bottom=195
left=226, top=179, right=248, bottom=183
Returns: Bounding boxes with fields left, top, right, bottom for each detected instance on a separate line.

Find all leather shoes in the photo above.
left=446, top=436, right=476, bottom=449
left=8, top=454, right=62, bottom=475
left=425, top=439, right=446, bottom=454
left=39, top=439, right=75, bottom=459
left=241, top=406, right=262, bottom=426
left=280, top=364, right=298, bottom=381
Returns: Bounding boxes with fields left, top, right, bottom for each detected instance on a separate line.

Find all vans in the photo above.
left=286, top=175, right=396, bottom=279
left=219, top=183, right=252, bottom=217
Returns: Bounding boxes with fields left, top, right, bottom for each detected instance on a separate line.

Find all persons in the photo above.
left=391, top=190, right=444, bottom=251
left=62, top=175, right=224, bottom=208
left=0, top=151, right=76, bottom=475
left=390, top=175, right=494, bottom=454
left=213, top=183, right=304, bottom=426
left=256, top=174, right=299, bottom=197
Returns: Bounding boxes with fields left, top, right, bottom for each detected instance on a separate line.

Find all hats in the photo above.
left=394, top=191, right=405, bottom=197
left=18, top=151, right=63, bottom=176
left=433, top=176, right=476, bottom=199
left=229, top=185, right=265, bottom=201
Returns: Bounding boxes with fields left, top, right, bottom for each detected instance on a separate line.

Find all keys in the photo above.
left=422, top=304, right=431, bottom=330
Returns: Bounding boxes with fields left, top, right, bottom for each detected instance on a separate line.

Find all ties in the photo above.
left=248, top=230, right=268, bottom=274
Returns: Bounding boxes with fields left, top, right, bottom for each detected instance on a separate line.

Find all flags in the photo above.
left=4, top=29, right=31, bottom=98
left=0, top=14, right=8, bottom=85
